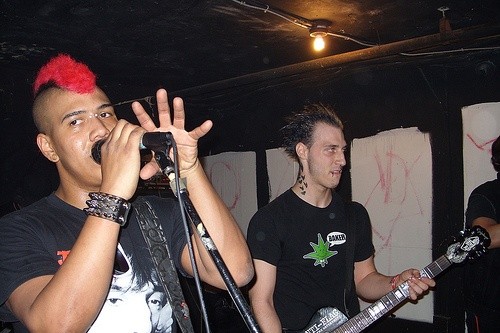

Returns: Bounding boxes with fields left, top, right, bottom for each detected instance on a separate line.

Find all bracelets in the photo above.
left=390, top=274, right=400, bottom=290
left=83, top=192, right=131, bottom=226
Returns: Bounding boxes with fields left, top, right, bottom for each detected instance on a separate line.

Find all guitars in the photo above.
left=281, top=224, right=491, bottom=333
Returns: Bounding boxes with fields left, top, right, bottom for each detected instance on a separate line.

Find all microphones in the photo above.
left=91, top=131, right=174, bottom=165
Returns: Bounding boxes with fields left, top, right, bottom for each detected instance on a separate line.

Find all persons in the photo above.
left=0, top=52, right=254, bottom=333
left=86, top=238, right=173, bottom=333
left=465, top=135, right=500, bottom=333
left=247, top=102, right=436, bottom=333
